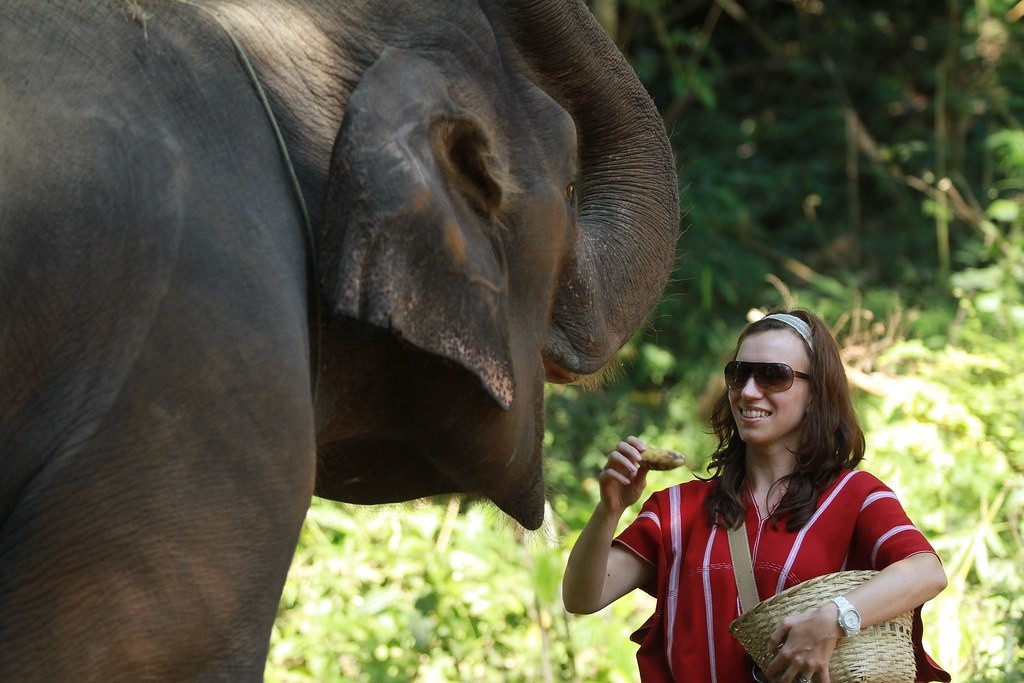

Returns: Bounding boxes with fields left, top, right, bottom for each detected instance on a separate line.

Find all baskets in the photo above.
left=729, top=571, right=917, bottom=683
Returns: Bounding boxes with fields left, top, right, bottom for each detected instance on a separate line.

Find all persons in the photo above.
left=562, top=307, right=950, bottom=683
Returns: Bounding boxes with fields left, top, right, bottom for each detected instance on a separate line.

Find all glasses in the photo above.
left=724, top=361, right=809, bottom=392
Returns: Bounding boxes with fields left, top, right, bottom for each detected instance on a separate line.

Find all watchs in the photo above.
left=829, top=591, right=865, bottom=640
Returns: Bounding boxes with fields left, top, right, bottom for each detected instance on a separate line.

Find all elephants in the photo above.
left=0, top=0, right=681, bottom=683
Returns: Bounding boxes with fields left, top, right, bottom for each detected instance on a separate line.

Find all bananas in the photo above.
left=635, top=447, right=686, bottom=470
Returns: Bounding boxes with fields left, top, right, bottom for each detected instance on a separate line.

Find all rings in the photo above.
left=800, top=677, right=815, bottom=683
